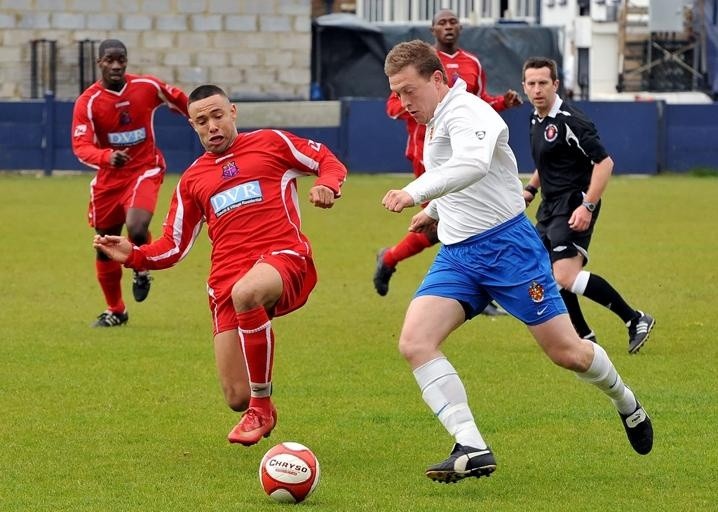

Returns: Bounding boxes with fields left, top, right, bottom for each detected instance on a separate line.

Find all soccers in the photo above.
left=260, top=442, right=320, bottom=503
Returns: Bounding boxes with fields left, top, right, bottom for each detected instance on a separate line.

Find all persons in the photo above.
left=72, top=37, right=188, bottom=328
left=375, top=9, right=523, bottom=296
left=379, top=39, right=653, bottom=481
left=523, top=56, right=657, bottom=354
left=91, top=85, right=348, bottom=447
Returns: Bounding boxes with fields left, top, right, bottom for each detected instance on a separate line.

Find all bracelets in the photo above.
left=525, top=185, right=538, bottom=196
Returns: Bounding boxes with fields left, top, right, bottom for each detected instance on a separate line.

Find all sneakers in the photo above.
left=374, top=248, right=396, bottom=295
left=625, top=310, right=655, bottom=353
left=612, top=383, right=653, bottom=454
left=132, top=270, right=151, bottom=301
left=228, top=405, right=277, bottom=445
left=91, top=306, right=128, bottom=327
left=425, top=442, right=496, bottom=483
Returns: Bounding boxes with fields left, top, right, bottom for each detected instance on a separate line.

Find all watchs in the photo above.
left=582, top=201, right=596, bottom=212
